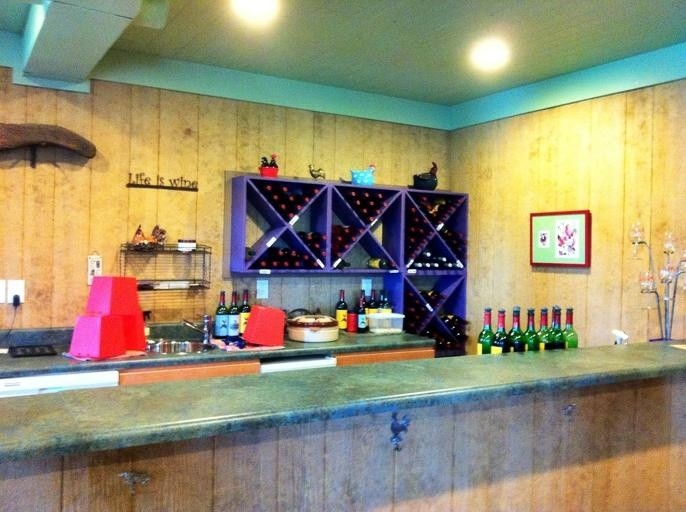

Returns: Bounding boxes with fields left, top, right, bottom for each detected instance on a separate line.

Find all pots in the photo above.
left=286, top=313, right=341, bottom=342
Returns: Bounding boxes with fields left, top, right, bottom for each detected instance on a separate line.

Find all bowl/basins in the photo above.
left=259, top=167, right=278, bottom=176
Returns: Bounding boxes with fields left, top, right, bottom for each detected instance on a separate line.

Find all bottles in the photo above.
left=200, top=289, right=250, bottom=344
left=337, top=289, right=393, bottom=332
left=246, top=186, right=467, bottom=271
left=407, top=289, right=469, bottom=350
left=478, top=305, right=577, bottom=355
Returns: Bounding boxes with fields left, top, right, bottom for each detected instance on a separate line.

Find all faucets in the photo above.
left=179, top=314, right=212, bottom=346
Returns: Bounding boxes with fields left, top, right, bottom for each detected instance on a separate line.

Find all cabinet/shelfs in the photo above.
left=231, top=175, right=469, bottom=358
left=120, top=243, right=212, bottom=291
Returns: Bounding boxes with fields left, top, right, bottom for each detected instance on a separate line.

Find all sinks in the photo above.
left=145, top=340, right=217, bottom=354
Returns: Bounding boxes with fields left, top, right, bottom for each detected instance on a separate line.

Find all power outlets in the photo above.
left=7, top=279, right=25, bottom=304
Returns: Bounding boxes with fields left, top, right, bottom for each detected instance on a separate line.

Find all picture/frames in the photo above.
left=530, top=210, right=591, bottom=269
